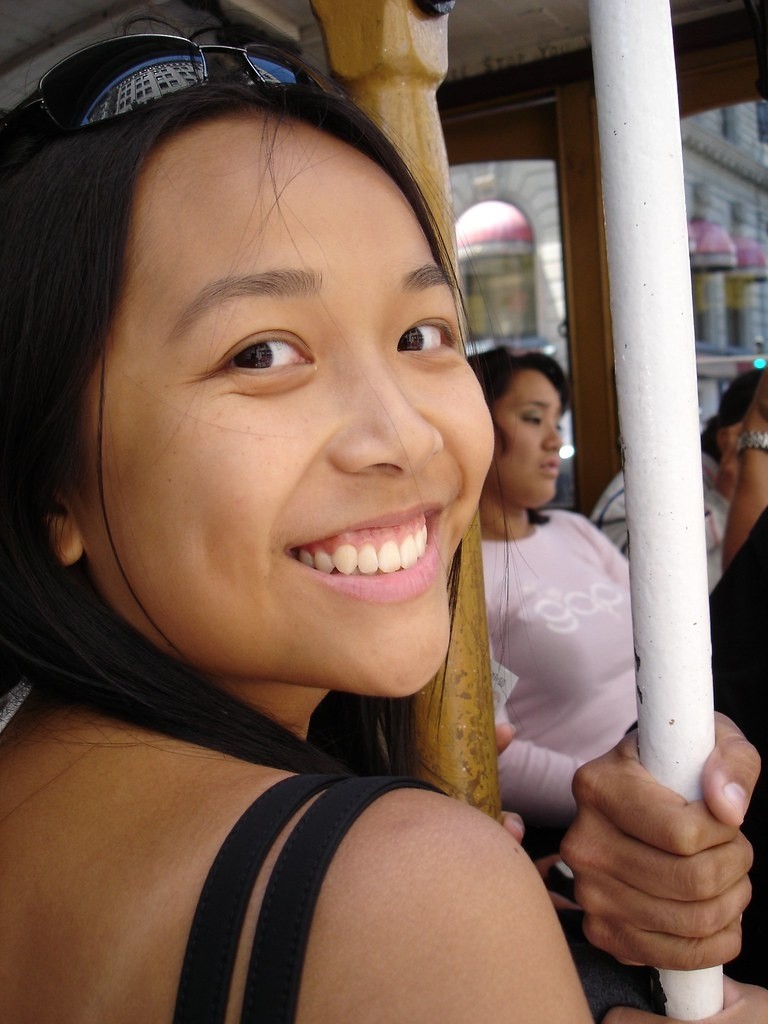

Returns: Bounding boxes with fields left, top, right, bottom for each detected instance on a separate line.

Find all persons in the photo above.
left=0, top=31, right=768, bottom=1023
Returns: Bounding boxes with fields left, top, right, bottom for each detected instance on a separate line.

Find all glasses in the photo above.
left=0, top=33, right=360, bottom=139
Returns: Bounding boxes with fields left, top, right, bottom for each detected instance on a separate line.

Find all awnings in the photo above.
left=731, top=235, right=768, bottom=284
left=455, top=201, right=534, bottom=263
left=686, top=219, right=737, bottom=273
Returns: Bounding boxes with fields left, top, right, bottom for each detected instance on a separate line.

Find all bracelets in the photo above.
left=736, top=429, right=767, bottom=451
